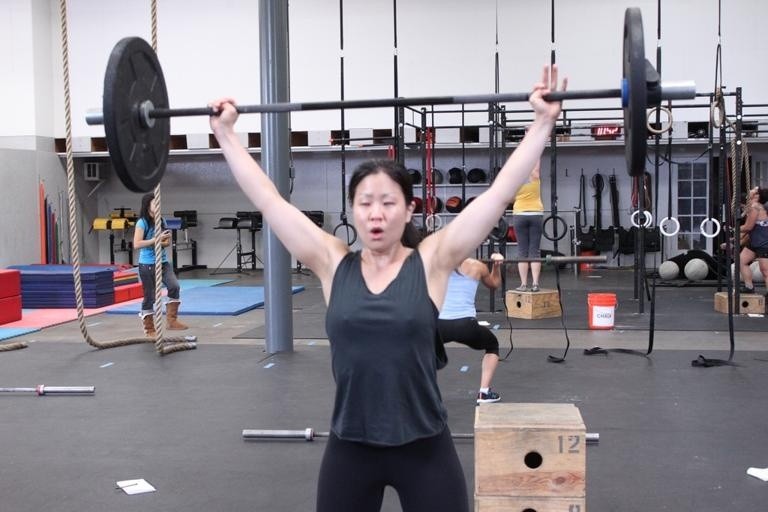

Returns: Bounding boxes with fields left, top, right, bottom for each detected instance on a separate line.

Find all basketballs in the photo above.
left=407, top=168, right=503, bottom=215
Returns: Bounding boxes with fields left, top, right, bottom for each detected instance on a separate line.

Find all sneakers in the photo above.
left=517, top=285, right=528, bottom=292
left=477, top=388, right=501, bottom=403
left=532, top=286, right=540, bottom=292
left=740, top=287, right=755, bottom=294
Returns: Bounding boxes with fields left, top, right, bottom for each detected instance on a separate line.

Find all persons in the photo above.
left=723, top=184, right=767, bottom=295
left=511, top=159, right=548, bottom=292
left=436, top=251, right=505, bottom=403
left=718, top=198, right=752, bottom=252
left=207, top=60, right=573, bottom=512
left=133, top=193, right=188, bottom=339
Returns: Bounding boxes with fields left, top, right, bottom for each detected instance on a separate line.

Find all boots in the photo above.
left=166, top=300, right=189, bottom=330
left=139, top=312, right=156, bottom=338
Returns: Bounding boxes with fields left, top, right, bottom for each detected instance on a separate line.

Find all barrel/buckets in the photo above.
left=587, top=293, right=618, bottom=329
left=580, top=251, right=595, bottom=271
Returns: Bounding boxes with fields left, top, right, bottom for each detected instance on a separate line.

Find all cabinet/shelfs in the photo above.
left=402, top=177, right=534, bottom=247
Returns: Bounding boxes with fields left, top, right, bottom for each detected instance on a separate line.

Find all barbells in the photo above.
left=86, top=7, right=696, bottom=193
